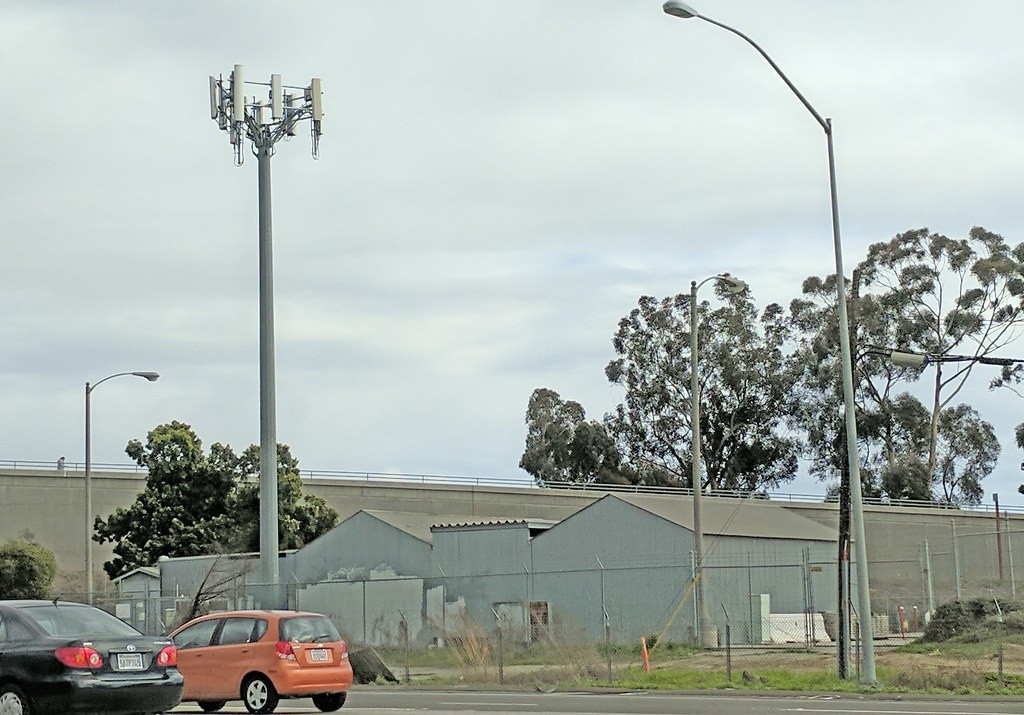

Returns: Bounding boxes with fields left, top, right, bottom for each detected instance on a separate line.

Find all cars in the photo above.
left=166, top=609, right=353, bottom=715
left=0, top=591, right=183, bottom=715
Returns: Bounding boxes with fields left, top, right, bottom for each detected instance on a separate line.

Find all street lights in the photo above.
left=210, top=64, right=326, bottom=609
left=84, top=371, right=160, bottom=606
left=690, top=274, right=744, bottom=650
left=662, top=1, right=876, bottom=687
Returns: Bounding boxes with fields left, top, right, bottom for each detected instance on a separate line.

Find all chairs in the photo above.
left=80, top=622, right=101, bottom=632
left=223, top=630, right=248, bottom=643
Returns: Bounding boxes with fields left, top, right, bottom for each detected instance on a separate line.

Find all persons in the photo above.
left=58, top=457, right=65, bottom=470
